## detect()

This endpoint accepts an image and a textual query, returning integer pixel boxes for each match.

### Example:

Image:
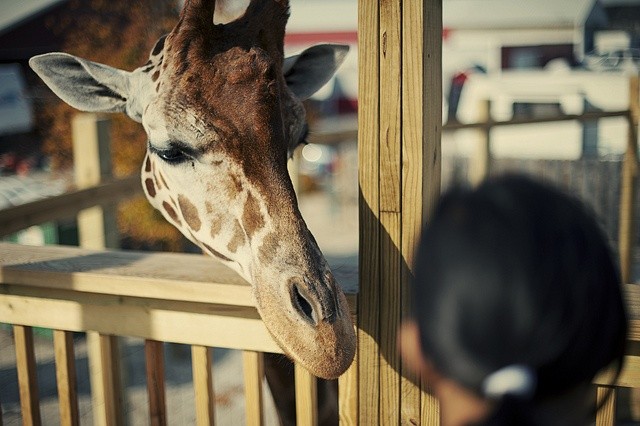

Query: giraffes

[0,0,355,426]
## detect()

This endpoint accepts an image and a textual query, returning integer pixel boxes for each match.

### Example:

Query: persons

[397,169,628,423]
